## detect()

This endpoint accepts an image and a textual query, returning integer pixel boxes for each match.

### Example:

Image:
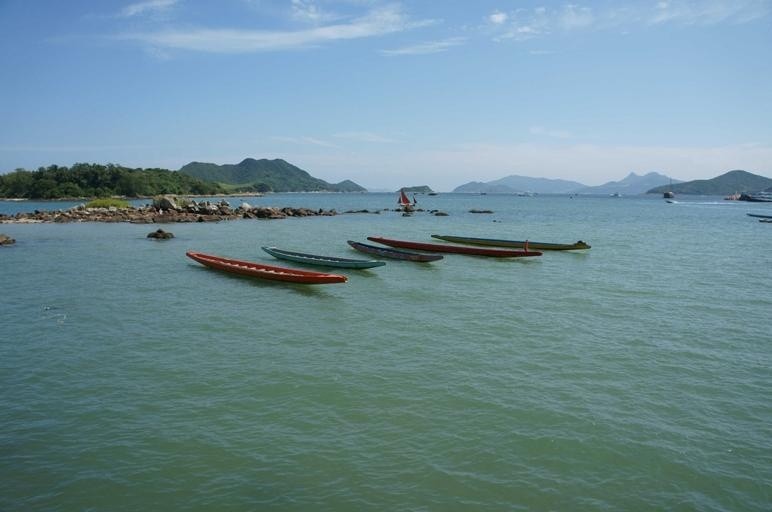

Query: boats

[366,235,543,261]
[259,244,387,271]
[431,233,591,253]
[184,248,349,286]
[345,240,444,264]
[663,191,674,198]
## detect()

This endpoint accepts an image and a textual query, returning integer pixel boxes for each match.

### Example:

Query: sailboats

[397,188,417,206]
